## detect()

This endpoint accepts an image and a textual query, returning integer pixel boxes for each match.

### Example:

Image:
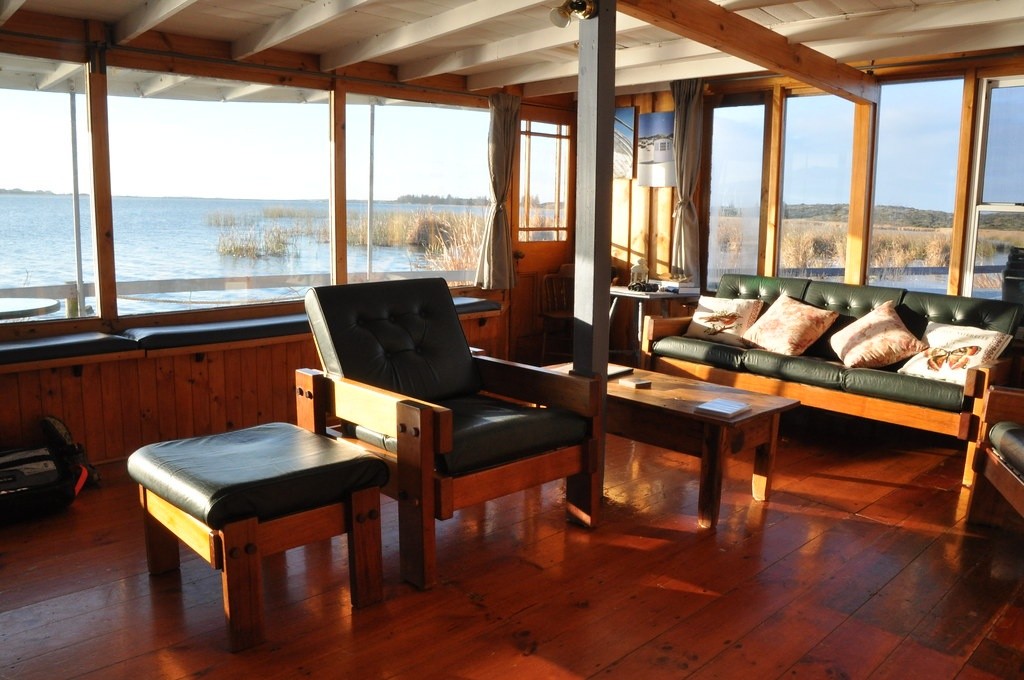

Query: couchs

[637,272,1024,488]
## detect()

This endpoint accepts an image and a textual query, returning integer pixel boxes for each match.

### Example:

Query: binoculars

[627,281,658,292]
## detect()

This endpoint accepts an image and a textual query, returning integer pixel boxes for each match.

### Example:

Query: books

[695,397,753,419]
[619,378,652,389]
[549,362,634,379]
[648,278,700,294]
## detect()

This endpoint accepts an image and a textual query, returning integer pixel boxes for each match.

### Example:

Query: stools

[126,420,390,658]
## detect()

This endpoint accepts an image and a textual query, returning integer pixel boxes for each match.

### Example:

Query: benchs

[1,292,502,482]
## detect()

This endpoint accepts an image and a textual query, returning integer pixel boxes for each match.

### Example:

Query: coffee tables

[539,359,803,529]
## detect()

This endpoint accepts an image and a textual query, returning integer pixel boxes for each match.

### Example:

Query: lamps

[548,0,599,28]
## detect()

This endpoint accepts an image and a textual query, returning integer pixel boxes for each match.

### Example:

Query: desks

[609,284,702,368]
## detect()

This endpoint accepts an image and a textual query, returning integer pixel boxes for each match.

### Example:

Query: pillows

[683,294,765,349]
[738,293,841,357]
[826,299,931,369]
[896,320,1014,386]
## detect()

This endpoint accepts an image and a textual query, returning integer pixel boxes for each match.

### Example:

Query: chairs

[952,385,1024,526]
[539,263,618,365]
[295,275,602,582]
